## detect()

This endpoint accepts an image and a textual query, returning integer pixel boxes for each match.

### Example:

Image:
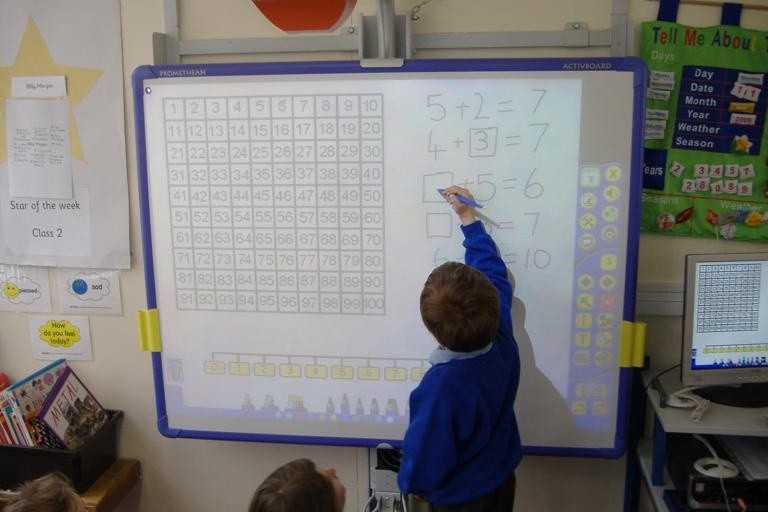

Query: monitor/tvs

[681,252,768,409]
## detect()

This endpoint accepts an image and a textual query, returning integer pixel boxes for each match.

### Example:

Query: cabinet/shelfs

[636,363,768,512]
[0,459,141,512]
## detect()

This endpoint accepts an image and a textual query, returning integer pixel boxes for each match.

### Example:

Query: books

[0,359,108,453]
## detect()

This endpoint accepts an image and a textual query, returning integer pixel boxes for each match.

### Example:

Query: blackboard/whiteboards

[134,58,648,460]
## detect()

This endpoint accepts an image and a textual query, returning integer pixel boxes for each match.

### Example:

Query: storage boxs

[0,408,124,493]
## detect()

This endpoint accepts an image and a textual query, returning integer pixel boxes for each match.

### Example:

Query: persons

[396,186,523,511]
[0,473,88,512]
[248,456,346,512]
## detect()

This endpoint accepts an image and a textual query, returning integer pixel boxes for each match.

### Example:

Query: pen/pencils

[436,188,484,209]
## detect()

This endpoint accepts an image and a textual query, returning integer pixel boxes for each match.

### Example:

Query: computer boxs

[664,435,749,512]
